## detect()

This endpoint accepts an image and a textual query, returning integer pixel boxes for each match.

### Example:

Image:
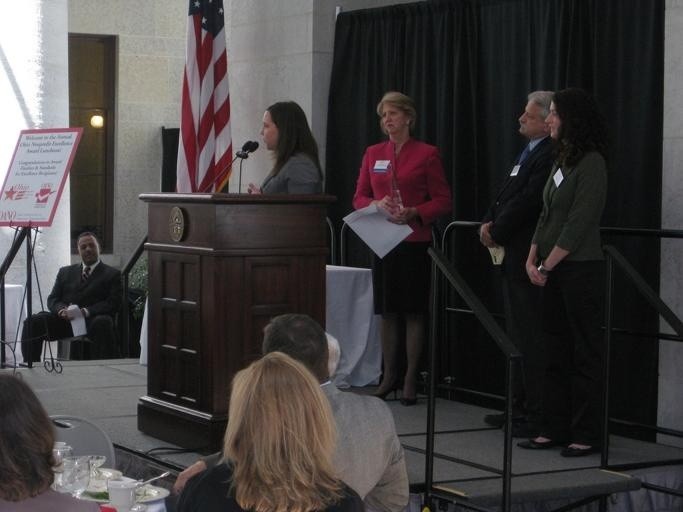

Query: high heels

[371,377,400,399]
[400,378,418,406]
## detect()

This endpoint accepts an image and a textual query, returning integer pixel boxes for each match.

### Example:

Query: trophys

[387,162,404,218]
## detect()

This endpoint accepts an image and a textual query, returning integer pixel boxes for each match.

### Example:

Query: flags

[176,0,232,193]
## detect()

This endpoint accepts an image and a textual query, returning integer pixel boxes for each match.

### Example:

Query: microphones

[200,141,253,192]
[239,141,260,195]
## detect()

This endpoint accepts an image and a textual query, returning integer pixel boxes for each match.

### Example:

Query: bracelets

[537,263,554,276]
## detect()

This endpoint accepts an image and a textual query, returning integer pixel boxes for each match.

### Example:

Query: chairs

[44,312,119,362]
[48,415,116,468]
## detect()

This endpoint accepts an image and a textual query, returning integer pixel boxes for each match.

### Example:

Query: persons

[258,316,409,512]
[21,231,122,361]
[516,89,611,458]
[353,91,451,408]
[247,102,323,196]
[1,374,102,511]
[173,332,341,495]
[480,89,558,437]
[172,352,365,511]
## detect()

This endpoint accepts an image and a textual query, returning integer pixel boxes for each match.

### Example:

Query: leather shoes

[561,442,598,458]
[485,408,524,426]
[517,434,559,450]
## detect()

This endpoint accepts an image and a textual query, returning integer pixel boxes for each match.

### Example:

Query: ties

[81,267,91,282]
[518,145,530,163]
